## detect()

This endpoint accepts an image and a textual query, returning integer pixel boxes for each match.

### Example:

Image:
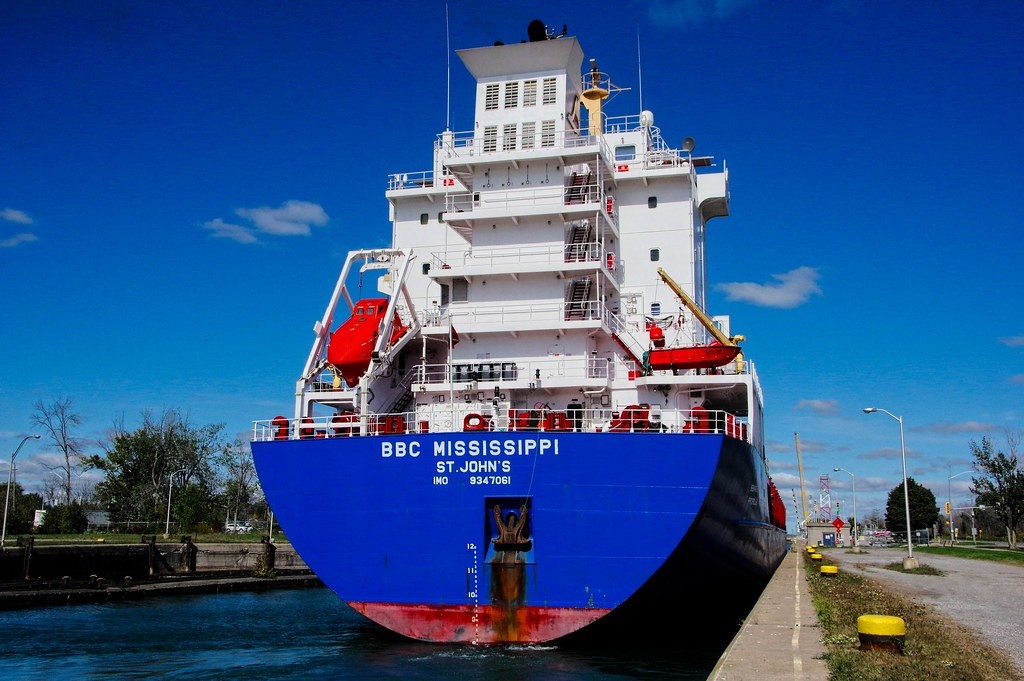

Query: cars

[247,527,253,533]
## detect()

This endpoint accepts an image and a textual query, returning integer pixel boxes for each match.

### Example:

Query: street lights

[164,469,188,538]
[948,470,975,541]
[834,468,858,547]
[863,407,912,557]
[2,434,40,544]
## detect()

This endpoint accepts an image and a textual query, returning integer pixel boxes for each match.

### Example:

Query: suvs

[227,524,247,533]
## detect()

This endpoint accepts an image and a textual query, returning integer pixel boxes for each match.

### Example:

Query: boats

[650,345,743,369]
[327,298,406,387]
[248,3,787,650]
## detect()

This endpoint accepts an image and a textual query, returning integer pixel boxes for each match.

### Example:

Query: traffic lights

[946,502,951,514]
